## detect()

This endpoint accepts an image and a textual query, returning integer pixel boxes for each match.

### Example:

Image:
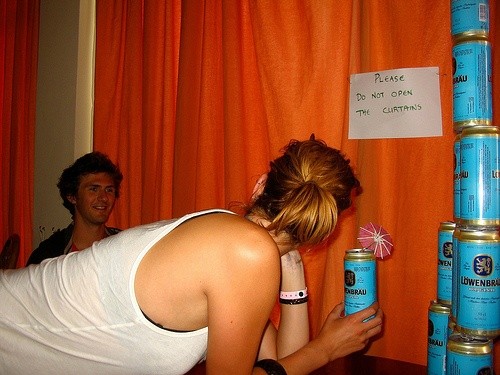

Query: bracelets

[279,287,308,300]
[279,296,308,304]
[254,359,287,375]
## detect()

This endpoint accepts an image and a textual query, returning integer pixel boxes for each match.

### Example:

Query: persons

[0,139,383,375]
[27,152,122,269]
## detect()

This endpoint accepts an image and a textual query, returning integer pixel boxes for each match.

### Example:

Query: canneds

[343,248,377,323]
[449,0,492,131]
[427,126,500,375]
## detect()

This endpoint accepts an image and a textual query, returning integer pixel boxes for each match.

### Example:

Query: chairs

[0,233,20,270]
